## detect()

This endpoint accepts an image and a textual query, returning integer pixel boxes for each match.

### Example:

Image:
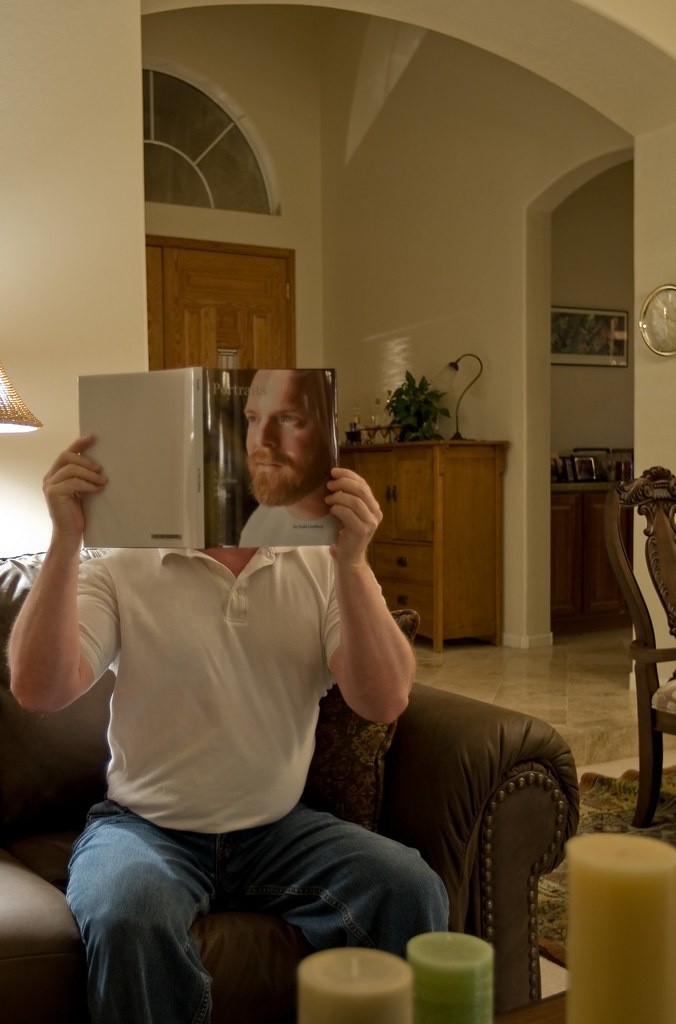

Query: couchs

[0,549,583,1024]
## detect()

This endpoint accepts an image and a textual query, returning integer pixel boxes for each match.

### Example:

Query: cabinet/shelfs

[550,481,634,637]
[338,437,507,653]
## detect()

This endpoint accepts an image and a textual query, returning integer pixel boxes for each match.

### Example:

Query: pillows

[304,608,423,830]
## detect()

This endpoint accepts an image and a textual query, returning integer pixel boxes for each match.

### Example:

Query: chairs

[601,462,676,830]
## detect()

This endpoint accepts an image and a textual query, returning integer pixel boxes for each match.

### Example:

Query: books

[74,367,340,549]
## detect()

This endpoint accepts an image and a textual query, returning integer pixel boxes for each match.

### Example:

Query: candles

[405,930,501,1024]
[296,946,415,1024]
[561,833,676,1024]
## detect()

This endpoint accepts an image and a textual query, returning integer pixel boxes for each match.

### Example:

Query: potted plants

[382,369,451,442]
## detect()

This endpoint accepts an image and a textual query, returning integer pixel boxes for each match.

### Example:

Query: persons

[240,369,339,546]
[9,433,450,1024]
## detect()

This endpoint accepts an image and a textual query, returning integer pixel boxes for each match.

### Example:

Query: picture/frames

[551,305,629,368]
[553,445,635,481]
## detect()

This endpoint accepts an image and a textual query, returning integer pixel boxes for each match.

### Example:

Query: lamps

[0,361,47,434]
[434,352,485,441]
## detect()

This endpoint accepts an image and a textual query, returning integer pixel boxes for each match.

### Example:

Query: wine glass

[364,414,402,445]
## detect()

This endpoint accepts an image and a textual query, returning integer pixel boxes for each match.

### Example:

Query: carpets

[536,762,676,973]
